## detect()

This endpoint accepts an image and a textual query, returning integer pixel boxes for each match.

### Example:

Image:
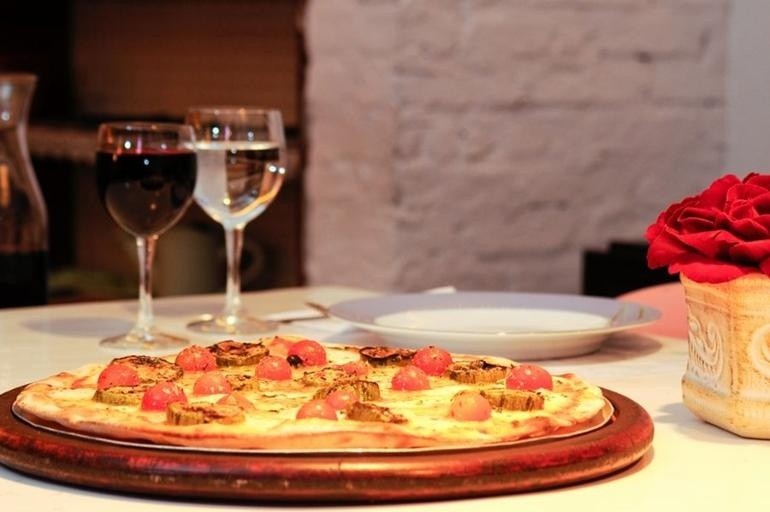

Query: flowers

[645,168,770,283]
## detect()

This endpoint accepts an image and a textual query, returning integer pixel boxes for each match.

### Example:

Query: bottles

[0,74,51,305]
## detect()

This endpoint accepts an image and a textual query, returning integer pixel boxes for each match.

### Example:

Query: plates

[327,288,664,361]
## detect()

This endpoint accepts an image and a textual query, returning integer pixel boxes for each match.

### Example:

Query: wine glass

[182,107,287,338]
[93,122,199,352]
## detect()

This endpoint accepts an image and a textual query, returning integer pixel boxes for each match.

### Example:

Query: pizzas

[13,332,607,452]
